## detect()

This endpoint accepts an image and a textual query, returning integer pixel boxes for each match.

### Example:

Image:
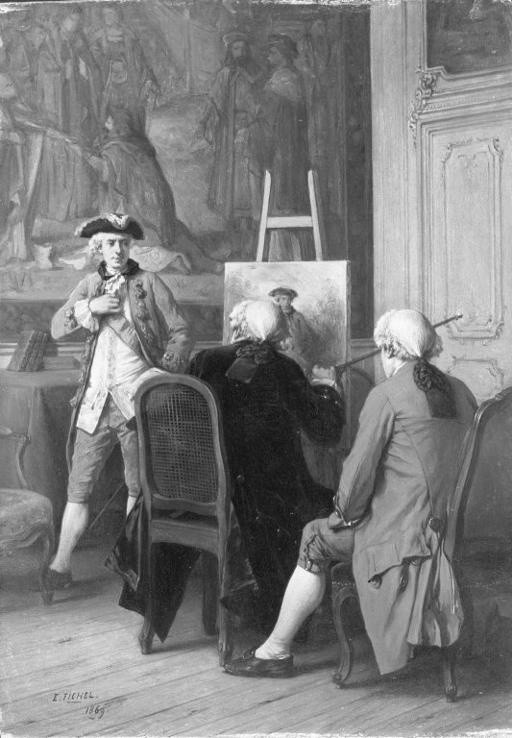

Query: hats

[75,212,143,239]
[268,287,298,297]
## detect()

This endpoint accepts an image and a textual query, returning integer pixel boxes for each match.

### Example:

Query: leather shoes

[32,568,72,592]
[224,648,293,677]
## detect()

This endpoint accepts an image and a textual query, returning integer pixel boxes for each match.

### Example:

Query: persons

[32,1,105,158]
[104,292,347,640]
[0,36,78,265]
[86,0,162,136]
[189,29,271,229]
[223,301,480,677]
[71,106,226,278]
[269,286,317,381]
[21,210,195,593]
[240,31,327,263]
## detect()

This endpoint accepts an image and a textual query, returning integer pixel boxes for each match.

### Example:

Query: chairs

[133,372,266,667]
[0,426,56,607]
[327,387,511,702]
